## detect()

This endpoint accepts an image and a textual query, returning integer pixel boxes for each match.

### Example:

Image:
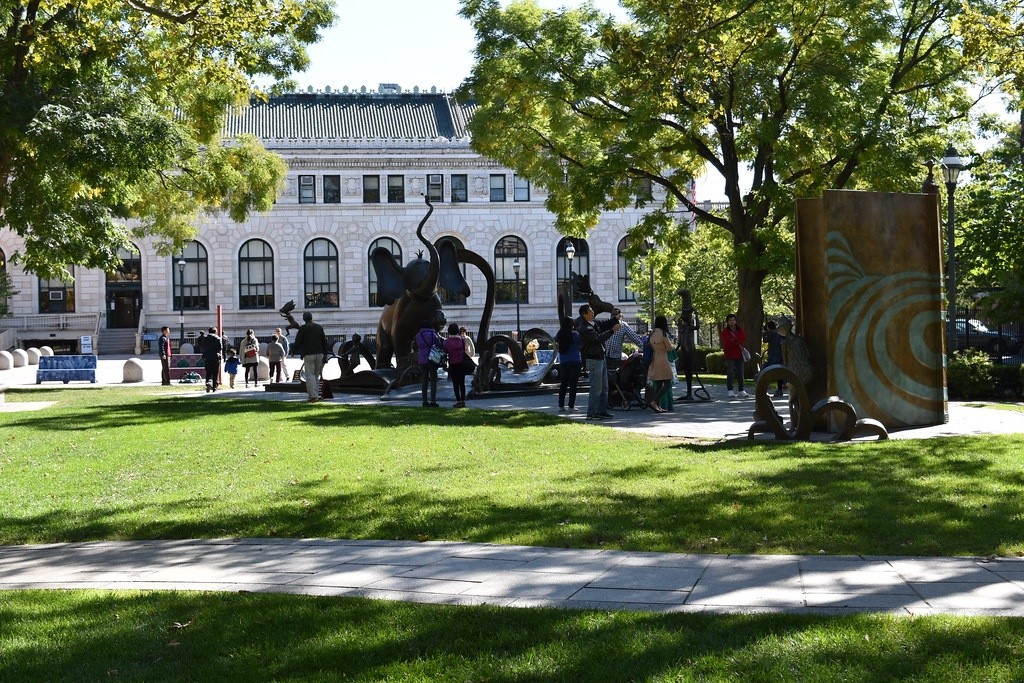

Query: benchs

[167,353,222,380]
[36,355,98,383]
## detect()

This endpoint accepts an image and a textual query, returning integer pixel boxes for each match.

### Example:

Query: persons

[773,312,814,432]
[195,330,206,353]
[578,304,624,420]
[239,328,261,388]
[720,314,749,398]
[276,328,290,382]
[280,312,301,336]
[291,312,328,402]
[416,319,475,407]
[225,349,241,389]
[265,335,285,384]
[201,326,223,394]
[555,315,582,411]
[645,315,679,413]
[222,331,229,363]
[524,337,539,366]
[763,321,786,399]
[342,333,361,377]
[603,309,644,374]
[158,326,172,385]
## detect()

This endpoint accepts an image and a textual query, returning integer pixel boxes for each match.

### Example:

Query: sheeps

[555,289,613,337]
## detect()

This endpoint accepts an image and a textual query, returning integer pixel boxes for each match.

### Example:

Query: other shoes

[213,388,216,393]
[558,407,566,412]
[207,385,210,393]
[567,407,579,412]
[771,392,783,398]
[453,401,465,407]
[430,402,439,407]
[660,408,667,412]
[423,402,430,407]
[308,398,319,403]
[599,412,612,419]
[586,413,603,420]
[650,402,662,412]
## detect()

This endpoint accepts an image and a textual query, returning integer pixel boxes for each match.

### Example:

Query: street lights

[565,240,576,319]
[939,141,960,367]
[644,224,657,330]
[511,257,521,341]
[177,256,187,348]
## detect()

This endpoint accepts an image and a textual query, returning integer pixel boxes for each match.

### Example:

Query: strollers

[606,350,648,411]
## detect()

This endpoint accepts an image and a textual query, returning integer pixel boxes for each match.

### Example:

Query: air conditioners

[49,291,63,300]
[301,176,313,185]
[430,174,441,184]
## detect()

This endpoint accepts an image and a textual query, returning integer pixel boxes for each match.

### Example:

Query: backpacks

[245,338,257,357]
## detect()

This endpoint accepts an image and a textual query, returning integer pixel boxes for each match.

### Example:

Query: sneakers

[738,391,749,397]
[728,391,735,397]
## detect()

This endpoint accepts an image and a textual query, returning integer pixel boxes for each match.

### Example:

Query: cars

[944,318,1024,357]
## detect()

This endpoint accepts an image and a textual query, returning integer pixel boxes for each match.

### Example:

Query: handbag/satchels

[427,344,441,364]
[741,347,751,362]
[643,335,653,363]
[461,353,475,374]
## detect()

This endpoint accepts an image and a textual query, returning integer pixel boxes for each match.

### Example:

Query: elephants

[371,195,470,379]
